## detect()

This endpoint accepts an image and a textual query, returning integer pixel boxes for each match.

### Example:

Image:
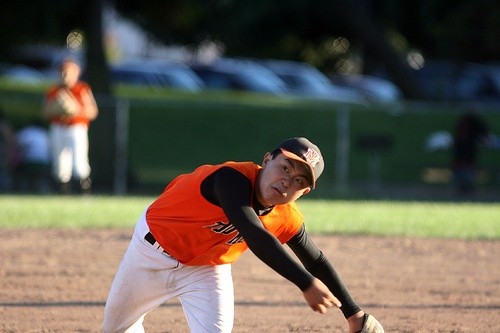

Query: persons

[0,26,493,195]
[101,137,384,333]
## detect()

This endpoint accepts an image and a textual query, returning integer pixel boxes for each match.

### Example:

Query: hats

[279,136,325,189]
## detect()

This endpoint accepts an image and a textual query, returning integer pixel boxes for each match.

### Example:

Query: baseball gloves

[56,86,80,117]
